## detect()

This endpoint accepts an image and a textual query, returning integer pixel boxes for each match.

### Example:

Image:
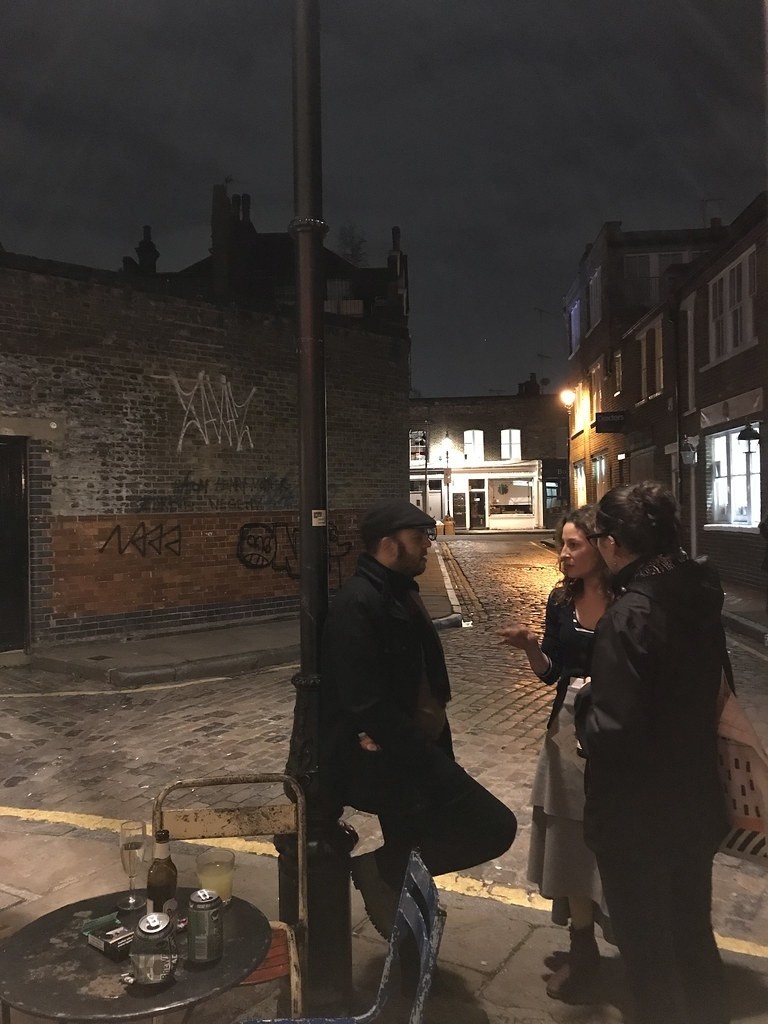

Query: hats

[358,497,436,549]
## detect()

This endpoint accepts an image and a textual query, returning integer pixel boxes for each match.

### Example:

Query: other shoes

[545,934,602,998]
[351,852,405,943]
[402,971,444,997]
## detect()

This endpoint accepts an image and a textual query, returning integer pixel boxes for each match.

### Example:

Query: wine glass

[115,820,146,911]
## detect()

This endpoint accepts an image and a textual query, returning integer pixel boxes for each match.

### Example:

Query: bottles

[147,831,178,938]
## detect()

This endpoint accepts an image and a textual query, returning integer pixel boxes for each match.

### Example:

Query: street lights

[441,432,453,520]
[560,389,576,517]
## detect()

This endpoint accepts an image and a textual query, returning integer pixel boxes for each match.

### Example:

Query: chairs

[235,847,447,1024]
[151,772,307,1023]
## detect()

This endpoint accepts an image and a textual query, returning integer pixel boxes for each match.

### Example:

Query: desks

[0,887,273,1024]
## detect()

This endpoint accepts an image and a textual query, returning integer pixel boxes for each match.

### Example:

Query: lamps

[680,433,696,465]
[737,419,768,454]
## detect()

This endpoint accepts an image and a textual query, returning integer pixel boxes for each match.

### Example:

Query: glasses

[586,533,620,550]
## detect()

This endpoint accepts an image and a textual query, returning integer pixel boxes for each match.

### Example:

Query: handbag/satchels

[713,689,768,868]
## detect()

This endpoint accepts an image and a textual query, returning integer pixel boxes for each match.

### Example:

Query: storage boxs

[87,924,135,963]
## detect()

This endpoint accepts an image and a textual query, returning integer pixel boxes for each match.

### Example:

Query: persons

[573,481,737,1024]
[495,505,618,1001]
[284,499,518,944]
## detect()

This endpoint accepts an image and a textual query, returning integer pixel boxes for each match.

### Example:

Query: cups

[194,850,234,905]
